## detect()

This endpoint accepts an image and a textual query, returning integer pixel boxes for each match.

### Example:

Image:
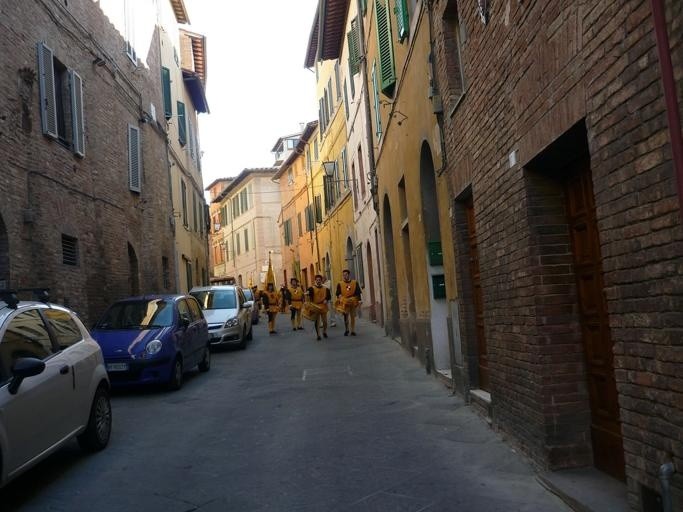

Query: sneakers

[323,333,328,338]
[318,336,321,340]
[351,332,356,336]
[344,331,349,336]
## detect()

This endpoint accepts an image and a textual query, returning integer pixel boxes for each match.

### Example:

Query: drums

[334,296,351,315]
[302,303,321,322]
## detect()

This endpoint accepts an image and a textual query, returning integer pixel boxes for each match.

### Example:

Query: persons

[337,270,362,336]
[251,275,337,341]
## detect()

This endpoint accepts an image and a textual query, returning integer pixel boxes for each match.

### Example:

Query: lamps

[320,157,359,195]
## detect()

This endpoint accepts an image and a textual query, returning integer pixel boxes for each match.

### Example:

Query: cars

[91,293,211,390]
[189,285,259,350]
[0,299,112,485]
